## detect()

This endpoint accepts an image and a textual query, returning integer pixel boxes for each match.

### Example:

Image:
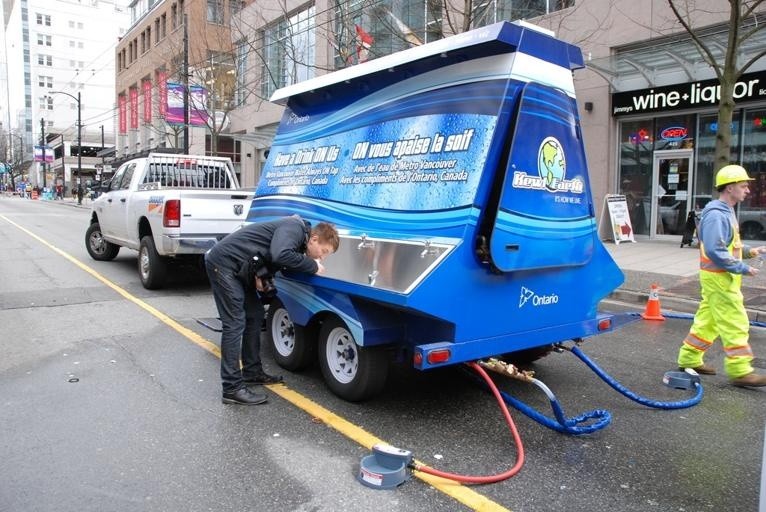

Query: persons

[677,164,766,388]
[0,161,106,207]
[203,214,341,406]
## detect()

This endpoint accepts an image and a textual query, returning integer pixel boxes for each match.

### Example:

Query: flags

[353,23,374,65]
[396,19,425,49]
[324,35,359,65]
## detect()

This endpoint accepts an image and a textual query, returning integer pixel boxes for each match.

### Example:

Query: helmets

[715,163,756,188]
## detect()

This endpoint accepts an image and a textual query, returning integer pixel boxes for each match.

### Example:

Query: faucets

[420,239,441,262]
[356,233,375,257]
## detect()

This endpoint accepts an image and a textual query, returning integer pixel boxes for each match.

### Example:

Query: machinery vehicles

[196,19,706,489]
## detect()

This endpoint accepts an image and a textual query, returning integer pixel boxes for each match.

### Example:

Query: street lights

[47,89,82,208]
[7,133,25,181]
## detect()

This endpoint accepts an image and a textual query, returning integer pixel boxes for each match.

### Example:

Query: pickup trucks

[86,154,254,290]
[660,196,766,239]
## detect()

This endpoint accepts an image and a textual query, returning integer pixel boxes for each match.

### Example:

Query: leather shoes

[240,370,286,385]
[221,387,268,406]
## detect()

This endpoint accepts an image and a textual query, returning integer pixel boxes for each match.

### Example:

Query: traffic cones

[639,282,665,322]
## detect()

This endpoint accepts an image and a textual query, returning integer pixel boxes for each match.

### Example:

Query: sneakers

[732,373,766,387]
[678,362,717,375]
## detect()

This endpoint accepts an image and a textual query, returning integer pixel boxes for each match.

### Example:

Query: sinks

[308,234,456,296]
